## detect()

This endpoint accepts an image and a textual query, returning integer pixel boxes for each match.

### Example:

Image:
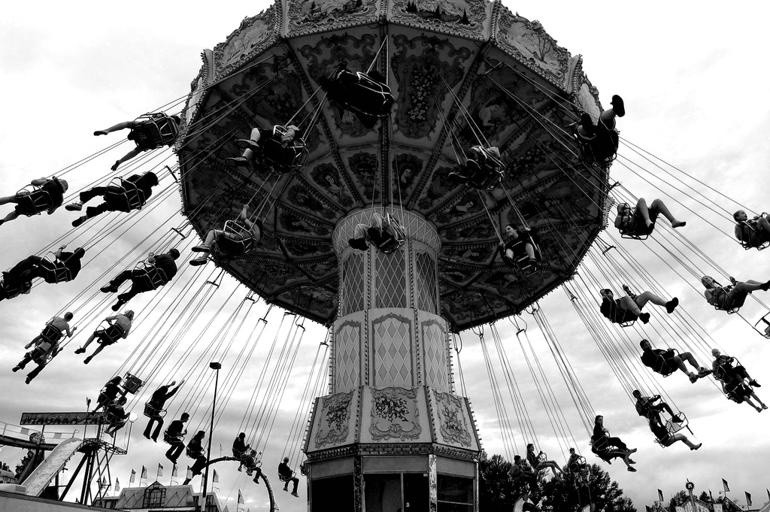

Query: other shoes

[237,467,298,498]
[90,409,202,476]
[12,346,92,385]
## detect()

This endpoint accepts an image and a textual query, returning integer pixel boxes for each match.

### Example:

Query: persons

[92,113,182,170]
[598,285,679,324]
[222,123,300,170]
[735,209,770,249]
[572,94,625,166]
[190,204,262,265]
[498,224,541,275]
[0,177,68,226]
[615,199,686,237]
[701,276,770,310]
[448,143,507,190]
[65,172,159,227]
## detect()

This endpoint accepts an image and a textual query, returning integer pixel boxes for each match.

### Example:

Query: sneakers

[762,280,770,291]
[112,160,119,171]
[647,220,654,230]
[581,110,596,135]
[112,299,123,312]
[189,257,208,265]
[236,136,262,152]
[93,129,108,136]
[528,415,703,493]
[65,203,82,211]
[101,285,117,294]
[641,312,651,324]
[612,94,625,118]
[448,171,469,184]
[748,379,760,387]
[528,258,538,268]
[224,156,250,169]
[699,369,714,378]
[671,220,686,229]
[666,296,680,313]
[466,158,483,176]
[192,244,212,254]
[502,256,518,271]
[72,216,87,227]
[689,372,700,382]
[756,402,768,412]
